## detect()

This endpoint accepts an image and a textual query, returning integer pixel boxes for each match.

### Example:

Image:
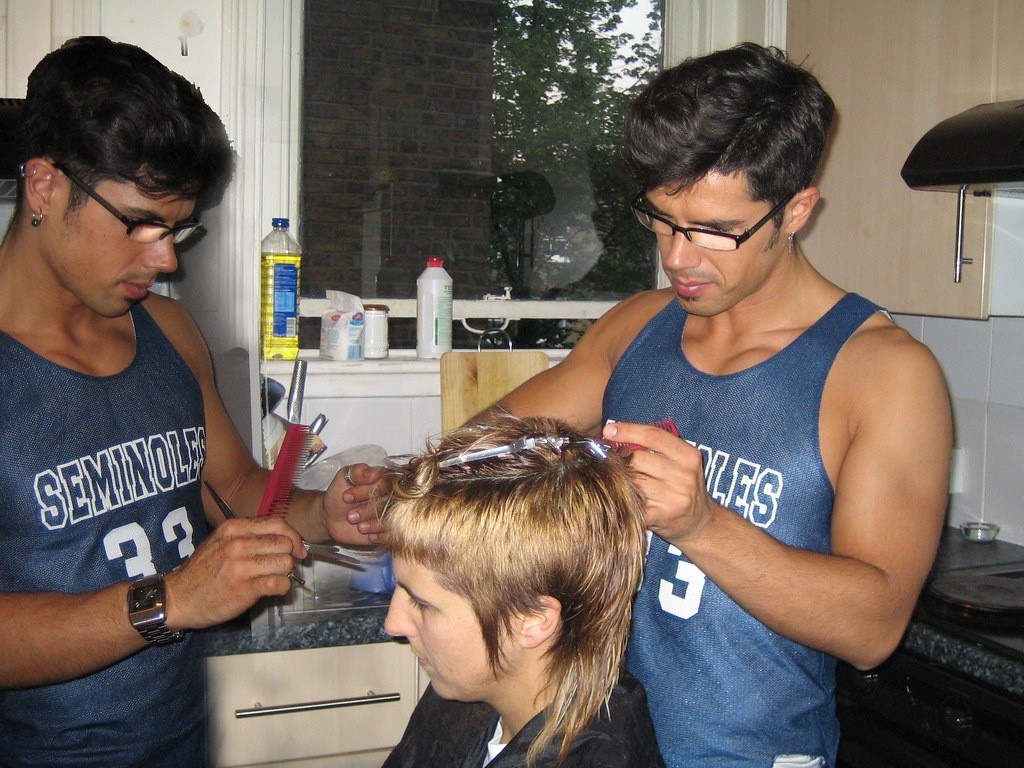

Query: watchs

[127,574,184,646]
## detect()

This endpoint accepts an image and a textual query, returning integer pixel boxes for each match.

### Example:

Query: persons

[458,43,952,767]
[380,412,664,768]
[0,36,395,768]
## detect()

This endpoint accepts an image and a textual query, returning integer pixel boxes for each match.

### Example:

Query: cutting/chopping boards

[439,329,549,438]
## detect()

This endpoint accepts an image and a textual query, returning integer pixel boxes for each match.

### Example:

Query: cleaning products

[416,258,453,360]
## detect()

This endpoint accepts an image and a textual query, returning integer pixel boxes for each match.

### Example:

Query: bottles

[415,256,455,361]
[260,217,302,361]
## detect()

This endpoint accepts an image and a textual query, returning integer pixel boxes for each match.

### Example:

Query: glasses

[53,159,203,246]
[631,184,811,253]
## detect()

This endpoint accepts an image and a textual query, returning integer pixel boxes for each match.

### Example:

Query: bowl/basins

[960,522,1000,544]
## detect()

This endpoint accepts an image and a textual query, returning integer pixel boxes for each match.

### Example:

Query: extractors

[899,99,1024,284]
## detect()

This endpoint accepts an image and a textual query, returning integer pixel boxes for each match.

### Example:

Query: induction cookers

[836,569,1024,768]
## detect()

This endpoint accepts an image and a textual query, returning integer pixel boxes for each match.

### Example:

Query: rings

[344,465,358,486]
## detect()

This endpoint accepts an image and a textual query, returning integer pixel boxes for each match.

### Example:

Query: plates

[931,569,1024,616]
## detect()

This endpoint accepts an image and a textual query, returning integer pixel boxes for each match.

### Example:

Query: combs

[259,425,315,519]
[602,419,679,456]
